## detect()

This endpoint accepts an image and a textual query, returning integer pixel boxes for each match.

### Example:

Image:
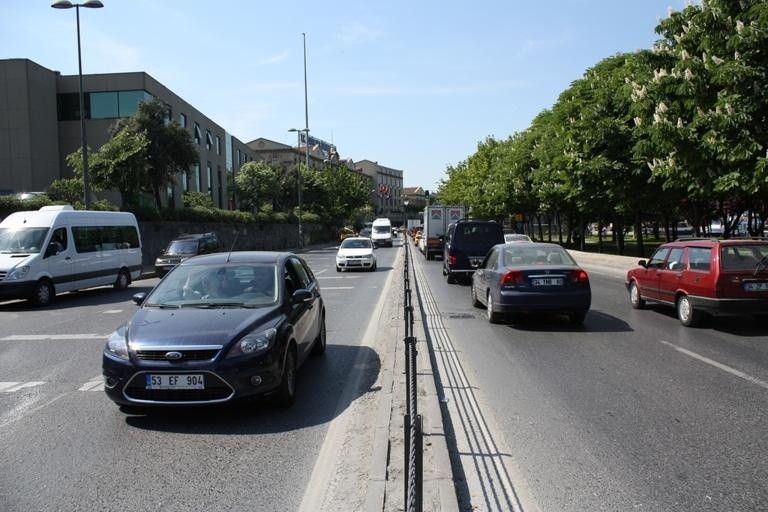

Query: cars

[101,250,327,411]
[589,216,762,236]
[335,237,378,273]
[471,242,591,325]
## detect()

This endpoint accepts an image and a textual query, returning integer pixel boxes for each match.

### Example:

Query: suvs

[154,233,220,280]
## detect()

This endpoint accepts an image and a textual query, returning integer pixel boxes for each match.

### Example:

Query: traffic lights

[425,190,429,199]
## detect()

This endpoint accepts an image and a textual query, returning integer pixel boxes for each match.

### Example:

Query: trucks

[356,218,398,249]
[408,206,536,284]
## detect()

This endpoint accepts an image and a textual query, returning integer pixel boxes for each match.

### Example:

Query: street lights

[50,1,104,211]
[288,128,310,250]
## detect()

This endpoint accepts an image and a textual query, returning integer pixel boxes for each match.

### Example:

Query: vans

[625,237,768,327]
[0,204,143,307]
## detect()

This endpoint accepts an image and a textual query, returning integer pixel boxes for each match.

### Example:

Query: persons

[194,266,233,301]
[239,266,282,301]
[40,232,65,252]
